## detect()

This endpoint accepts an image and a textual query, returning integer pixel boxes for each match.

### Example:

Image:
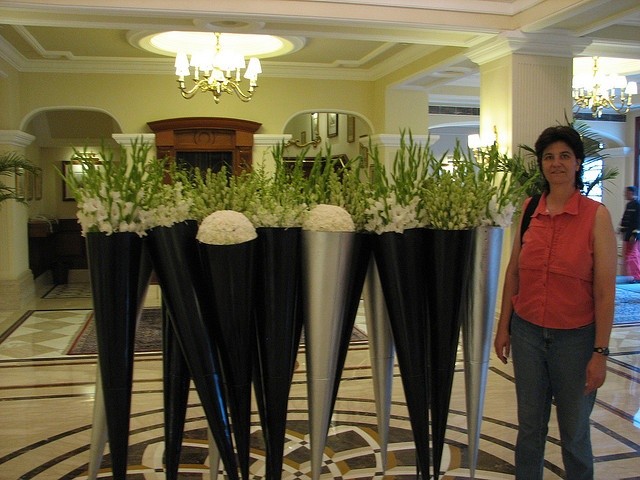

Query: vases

[87,238,156,480]
[427,229,475,480]
[83,232,142,479]
[461,227,503,479]
[161,289,190,480]
[194,236,256,480]
[362,252,394,475]
[146,220,240,480]
[249,227,304,480]
[327,230,376,432]
[304,232,372,479]
[372,228,430,479]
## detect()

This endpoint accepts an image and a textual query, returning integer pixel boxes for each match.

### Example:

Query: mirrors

[282,109,375,195]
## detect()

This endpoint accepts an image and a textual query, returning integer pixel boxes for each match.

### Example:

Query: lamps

[571,56,639,119]
[466,125,497,164]
[174,32,264,104]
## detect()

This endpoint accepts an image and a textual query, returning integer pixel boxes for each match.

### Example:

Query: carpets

[610,274,640,327]
[60,308,370,355]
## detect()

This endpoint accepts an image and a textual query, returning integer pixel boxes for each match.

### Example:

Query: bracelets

[593,347,610,356]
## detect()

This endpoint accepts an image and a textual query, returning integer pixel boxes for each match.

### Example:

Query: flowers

[299,139,373,232]
[49,136,150,239]
[298,204,355,232]
[257,137,308,227]
[448,139,541,229]
[193,209,258,245]
[362,126,450,234]
[190,158,274,227]
[420,169,499,232]
[140,153,196,233]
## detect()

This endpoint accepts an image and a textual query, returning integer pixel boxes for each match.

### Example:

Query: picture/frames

[27,166,34,201]
[35,170,44,200]
[327,113,338,138]
[346,115,355,143]
[311,112,319,141]
[15,165,27,200]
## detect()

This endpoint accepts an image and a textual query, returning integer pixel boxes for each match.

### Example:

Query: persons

[616,186,640,282]
[493,126,617,480]
